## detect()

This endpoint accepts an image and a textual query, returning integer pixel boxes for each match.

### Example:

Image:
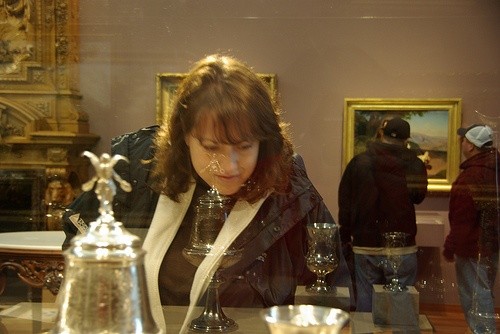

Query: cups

[467,252,500,334]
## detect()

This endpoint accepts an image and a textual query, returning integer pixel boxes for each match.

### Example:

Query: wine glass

[305,222,340,294]
[383,231,409,292]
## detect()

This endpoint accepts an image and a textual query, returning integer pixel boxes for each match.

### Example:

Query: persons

[63,55,352,306]
[338,119,429,312]
[442,125,500,334]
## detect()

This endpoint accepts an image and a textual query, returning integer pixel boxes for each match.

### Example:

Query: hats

[456,124,494,148]
[384,119,411,139]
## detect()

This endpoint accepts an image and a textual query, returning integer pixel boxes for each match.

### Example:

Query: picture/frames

[342,98,463,193]
[154,72,278,126]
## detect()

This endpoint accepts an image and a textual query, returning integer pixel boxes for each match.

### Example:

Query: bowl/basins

[258,303,351,334]
